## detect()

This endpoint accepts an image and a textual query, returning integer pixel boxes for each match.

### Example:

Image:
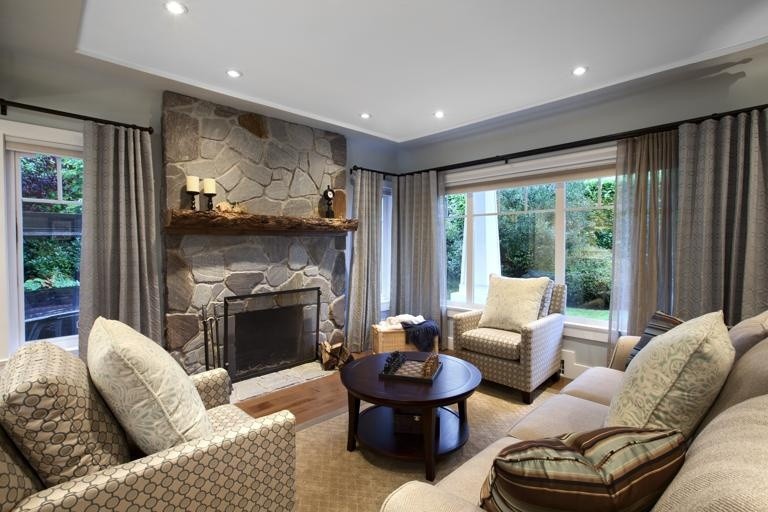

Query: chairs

[0,313,298,512]
[453,273,570,404]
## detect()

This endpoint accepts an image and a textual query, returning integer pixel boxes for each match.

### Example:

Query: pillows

[607,309,737,440]
[479,273,554,334]
[623,310,686,370]
[480,428,689,512]
[83,314,220,459]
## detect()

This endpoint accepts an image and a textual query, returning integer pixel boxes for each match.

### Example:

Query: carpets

[277,385,565,512]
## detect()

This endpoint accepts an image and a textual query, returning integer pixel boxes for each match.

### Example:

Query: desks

[339,351,473,482]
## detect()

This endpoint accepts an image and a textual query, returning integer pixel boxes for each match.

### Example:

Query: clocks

[323,184,335,218]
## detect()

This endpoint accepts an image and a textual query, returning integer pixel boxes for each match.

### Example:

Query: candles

[185,175,217,194]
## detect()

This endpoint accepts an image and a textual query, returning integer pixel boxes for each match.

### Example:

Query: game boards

[378,359,442,384]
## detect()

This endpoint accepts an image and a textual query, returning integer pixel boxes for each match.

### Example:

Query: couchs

[377,307,768,512]
